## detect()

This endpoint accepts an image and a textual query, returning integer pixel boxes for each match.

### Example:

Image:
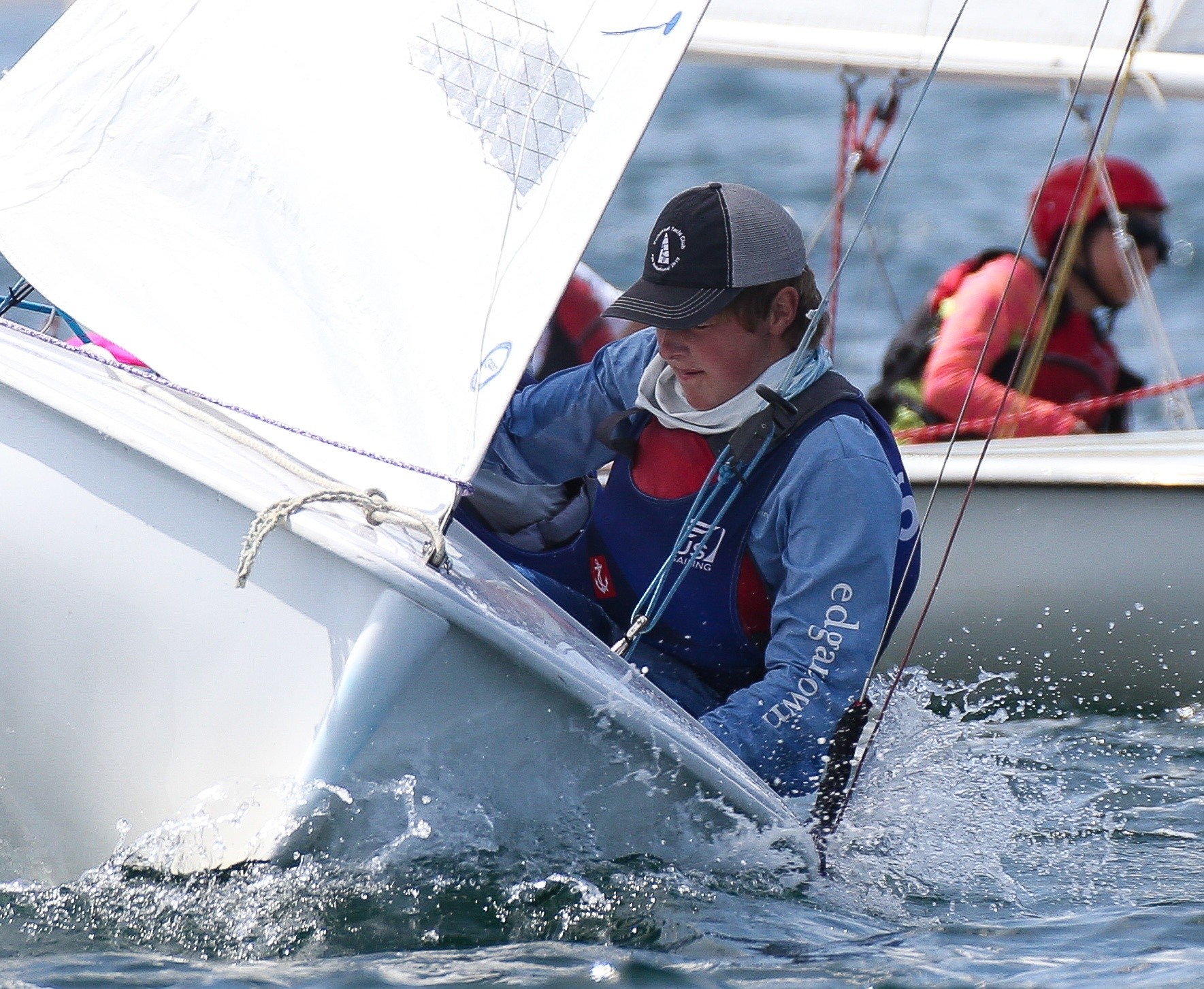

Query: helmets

[1030,154,1167,258]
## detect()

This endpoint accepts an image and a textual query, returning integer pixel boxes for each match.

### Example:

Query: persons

[455,181,920,796]
[866,153,1172,446]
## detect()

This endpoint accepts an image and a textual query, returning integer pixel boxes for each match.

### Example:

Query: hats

[600,183,807,331]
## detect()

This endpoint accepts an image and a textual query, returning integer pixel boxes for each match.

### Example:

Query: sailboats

[670,0,1203,719]
[1,0,834,902]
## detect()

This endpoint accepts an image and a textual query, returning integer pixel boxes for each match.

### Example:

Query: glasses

[1102,217,1171,266]
